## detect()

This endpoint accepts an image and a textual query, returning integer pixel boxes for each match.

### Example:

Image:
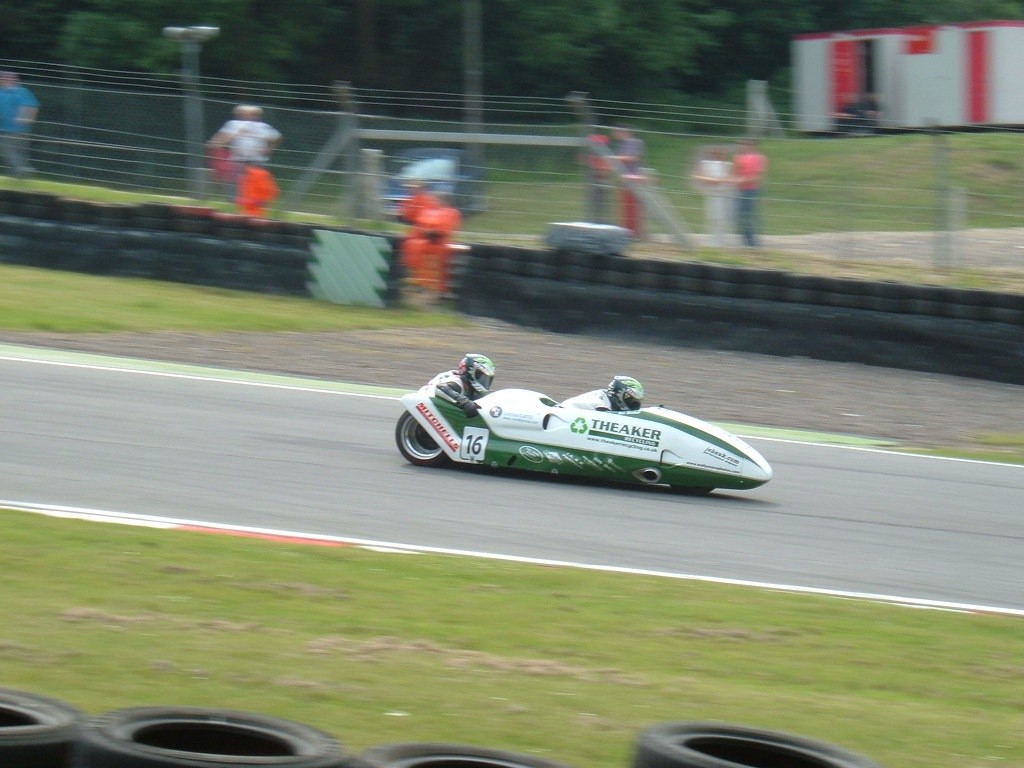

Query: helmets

[459,353,495,396]
[606,375,644,411]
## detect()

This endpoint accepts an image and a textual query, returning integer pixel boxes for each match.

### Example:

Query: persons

[550,375,646,413]
[690,134,770,246]
[586,125,646,238]
[830,92,883,137]
[209,103,284,208]
[396,183,438,286]
[423,352,496,417]
[0,68,39,178]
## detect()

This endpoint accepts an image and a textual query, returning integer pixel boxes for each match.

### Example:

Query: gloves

[456,397,482,418]
